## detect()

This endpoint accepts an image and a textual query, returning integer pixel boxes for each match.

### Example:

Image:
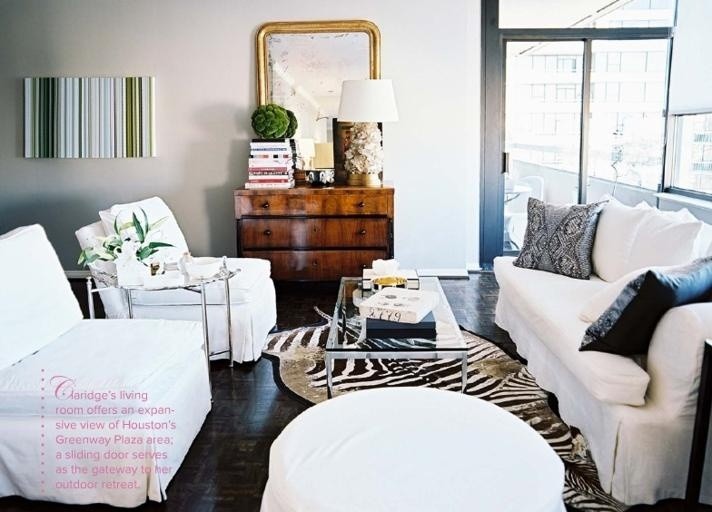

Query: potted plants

[76,205,176,286]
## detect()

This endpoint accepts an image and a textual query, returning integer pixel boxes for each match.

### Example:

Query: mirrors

[256,19,383,181]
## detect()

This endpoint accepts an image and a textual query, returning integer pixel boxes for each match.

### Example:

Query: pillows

[579,257,712,353]
[513,197,608,280]
[99,197,188,271]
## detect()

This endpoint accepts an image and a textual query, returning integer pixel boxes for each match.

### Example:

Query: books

[358,286,439,340]
[242,137,297,190]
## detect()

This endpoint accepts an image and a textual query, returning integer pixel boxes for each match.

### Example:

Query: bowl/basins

[372,277,408,295]
[184,256,222,278]
[304,169,336,186]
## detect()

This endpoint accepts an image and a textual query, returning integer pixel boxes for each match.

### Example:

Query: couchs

[492,196,712,508]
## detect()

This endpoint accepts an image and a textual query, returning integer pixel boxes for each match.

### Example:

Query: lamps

[337,78,397,189]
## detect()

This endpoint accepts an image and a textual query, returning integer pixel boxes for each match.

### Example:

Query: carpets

[259,314,624,510]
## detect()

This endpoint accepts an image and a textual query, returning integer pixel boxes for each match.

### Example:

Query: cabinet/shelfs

[235,181,397,285]
